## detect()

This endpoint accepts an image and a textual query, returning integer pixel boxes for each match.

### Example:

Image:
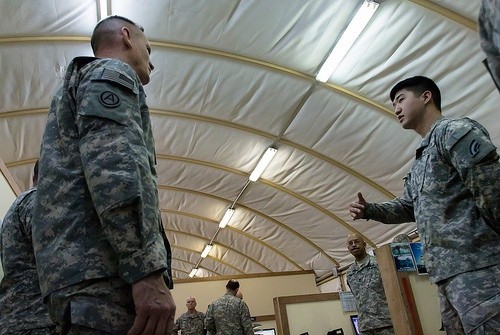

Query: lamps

[248,147,278,182]
[201,244,212,258]
[189,268,198,278]
[315,0,380,83]
[219,209,235,229]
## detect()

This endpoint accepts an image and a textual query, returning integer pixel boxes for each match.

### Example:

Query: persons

[206,279,254,335]
[33,16,176,335]
[0,163,55,335]
[477,0,500,90]
[346,234,394,335]
[348,75,500,335]
[173,297,207,335]
